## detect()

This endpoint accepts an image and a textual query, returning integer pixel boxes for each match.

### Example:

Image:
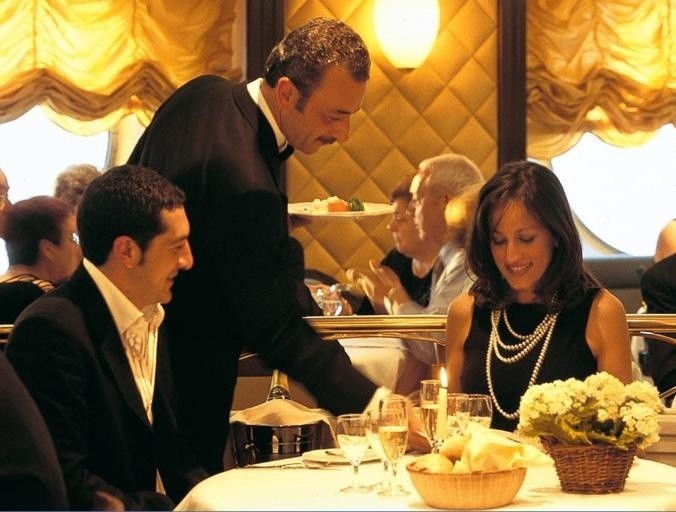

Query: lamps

[374,0,439,71]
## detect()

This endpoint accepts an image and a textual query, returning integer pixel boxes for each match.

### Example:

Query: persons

[6,166,196,511]
[345,177,437,315]
[357,153,486,400]
[0,162,102,339]
[398,161,632,454]
[127,13,391,506]
[640,217,676,407]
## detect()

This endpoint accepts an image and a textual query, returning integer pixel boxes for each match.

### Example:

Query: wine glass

[335,396,412,497]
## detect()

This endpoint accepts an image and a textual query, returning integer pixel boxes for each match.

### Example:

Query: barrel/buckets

[232,421,322,468]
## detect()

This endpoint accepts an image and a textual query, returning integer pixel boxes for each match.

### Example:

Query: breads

[414,435,524,474]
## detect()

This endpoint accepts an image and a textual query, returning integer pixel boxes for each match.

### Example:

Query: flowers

[516,372,661,446]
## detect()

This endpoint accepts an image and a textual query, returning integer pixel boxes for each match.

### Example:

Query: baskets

[540,436,644,495]
[406,453,527,510]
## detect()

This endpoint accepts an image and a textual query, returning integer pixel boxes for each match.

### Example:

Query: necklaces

[485,287,563,420]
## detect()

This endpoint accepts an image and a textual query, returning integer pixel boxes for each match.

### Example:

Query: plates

[286,201,397,219]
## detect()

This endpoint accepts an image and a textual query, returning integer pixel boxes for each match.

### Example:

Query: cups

[313,291,344,318]
[298,363,494,471]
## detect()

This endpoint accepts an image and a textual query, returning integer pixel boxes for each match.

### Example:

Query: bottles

[264,368,290,402]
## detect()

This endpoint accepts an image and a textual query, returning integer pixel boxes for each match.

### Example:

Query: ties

[432,258,445,293]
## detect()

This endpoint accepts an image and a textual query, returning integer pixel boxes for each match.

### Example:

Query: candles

[435,366,452,442]
